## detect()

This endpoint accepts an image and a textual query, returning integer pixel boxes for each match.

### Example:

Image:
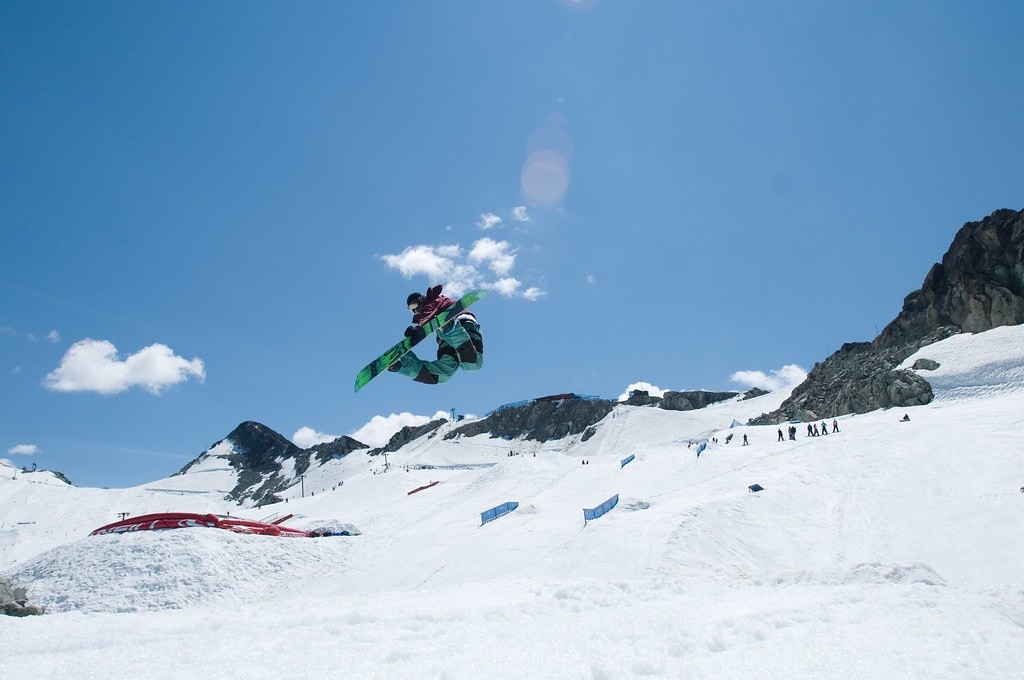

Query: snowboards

[352,288,490,393]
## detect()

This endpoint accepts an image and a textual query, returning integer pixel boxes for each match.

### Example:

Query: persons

[833,419,840,432]
[788,426,796,440]
[903,414,909,420]
[808,423,820,436]
[387,292,483,384]
[778,429,784,441]
[821,421,828,435]
[743,434,749,446]
[510,450,512,456]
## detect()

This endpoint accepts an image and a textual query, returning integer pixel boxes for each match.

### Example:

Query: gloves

[404,326,415,337]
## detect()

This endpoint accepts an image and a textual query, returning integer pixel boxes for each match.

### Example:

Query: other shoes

[443,316,458,335]
[387,360,402,372]
